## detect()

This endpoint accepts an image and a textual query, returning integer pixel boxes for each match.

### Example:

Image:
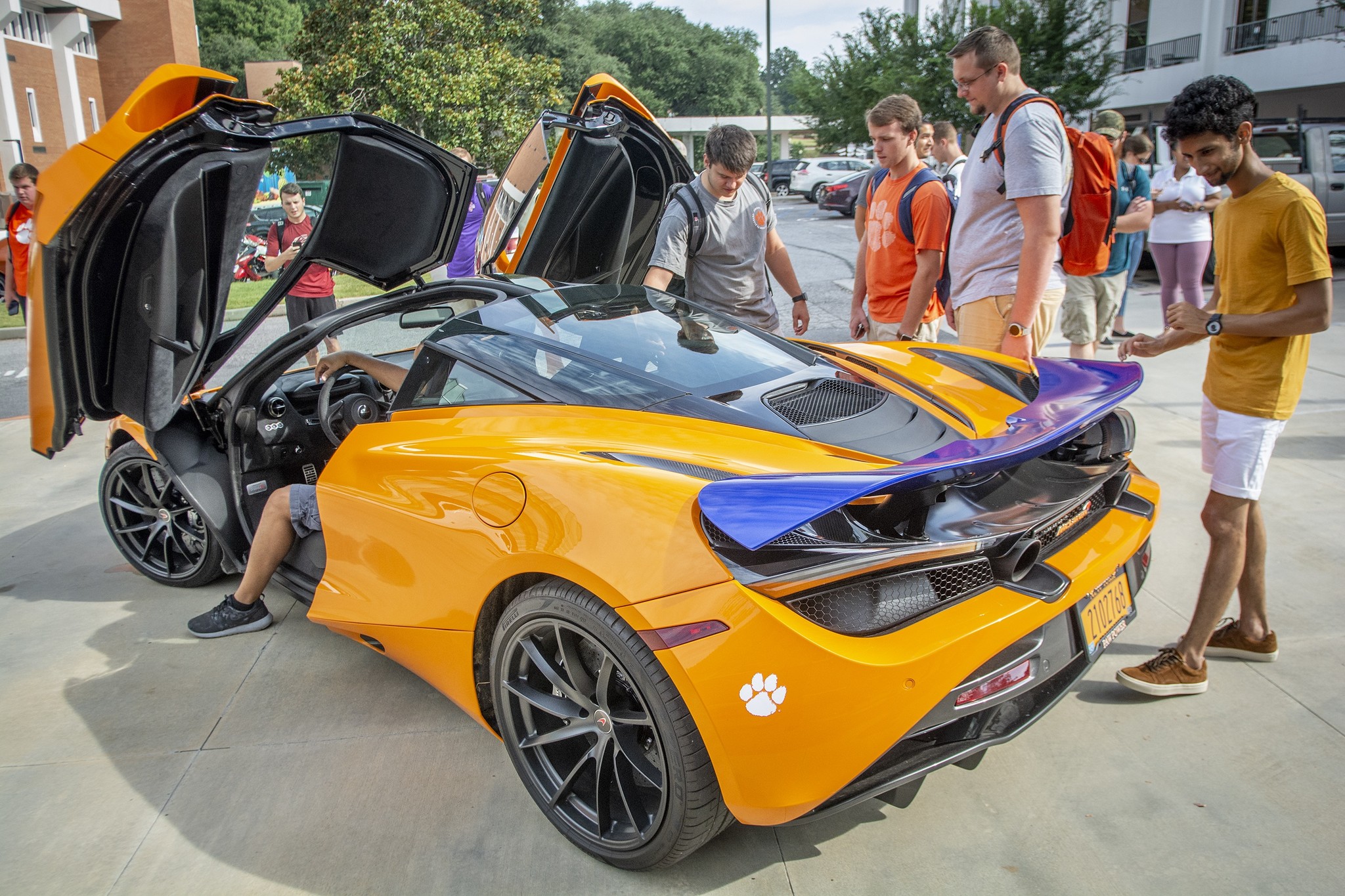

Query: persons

[642,127,813,351]
[644,288,742,355]
[853,117,973,251]
[263,183,342,369]
[1143,127,1223,331]
[1115,77,1331,698]
[849,93,954,350]
[1056,109,1158,377]
[0,163,46,333]
[445,147,496,279]
[188,329,468,640]
[936,26,1075,367]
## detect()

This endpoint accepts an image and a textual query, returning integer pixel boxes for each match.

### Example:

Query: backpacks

[870,167,951,310]
[979,93,1120,276]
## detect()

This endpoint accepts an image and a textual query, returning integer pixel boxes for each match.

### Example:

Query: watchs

[1004,321,1034,338]
[792,292,808,303]
[896,329,913,342]
[1205,313,1222,336]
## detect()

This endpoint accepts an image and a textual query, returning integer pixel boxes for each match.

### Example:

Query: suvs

[788,157,875,204]
[760,159,801,197]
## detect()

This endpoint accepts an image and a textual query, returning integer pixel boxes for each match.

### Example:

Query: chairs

[550,341,649,391]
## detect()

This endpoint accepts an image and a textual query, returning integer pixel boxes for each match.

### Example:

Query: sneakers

[1115,648,1208,696]
[186,592,274,638]
[1177,616,1279,662]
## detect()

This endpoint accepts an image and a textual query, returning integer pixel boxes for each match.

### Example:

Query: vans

[1203,122,1345,285]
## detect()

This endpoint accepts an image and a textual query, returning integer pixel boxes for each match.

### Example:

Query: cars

[240,201,323,242]
[749,161,767,175]
[816,168,872,218]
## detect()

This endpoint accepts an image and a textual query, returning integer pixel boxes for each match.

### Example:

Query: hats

[1093,109,1125,137]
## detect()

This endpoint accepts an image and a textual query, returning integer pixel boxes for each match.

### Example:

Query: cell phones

[8,301,19,315]
[293,242,301,251]
[1179,201,1192,208]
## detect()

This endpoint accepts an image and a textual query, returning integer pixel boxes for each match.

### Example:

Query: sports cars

[11,55,1167,872]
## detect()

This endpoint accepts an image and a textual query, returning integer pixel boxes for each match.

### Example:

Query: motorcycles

[232,222,285,283]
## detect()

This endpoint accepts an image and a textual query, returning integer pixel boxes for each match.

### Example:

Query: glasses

[1132,153,1147,164]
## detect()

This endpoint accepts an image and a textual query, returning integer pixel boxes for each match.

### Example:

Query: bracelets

[1199,204,1204,212]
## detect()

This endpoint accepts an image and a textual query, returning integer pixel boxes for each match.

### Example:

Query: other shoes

[1110,330,1135,339]
[1097,336,1114,348]
